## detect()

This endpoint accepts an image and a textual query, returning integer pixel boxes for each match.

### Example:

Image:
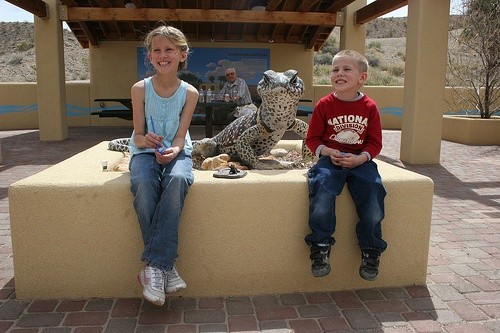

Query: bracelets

[234,96,237,101]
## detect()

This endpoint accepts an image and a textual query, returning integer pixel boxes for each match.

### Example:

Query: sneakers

[138,264,187,306]
[310,245,331,276]
[359,252,379,281]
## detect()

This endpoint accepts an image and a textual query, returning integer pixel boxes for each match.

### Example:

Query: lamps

[124,0,136,9]
[249,0,268,11]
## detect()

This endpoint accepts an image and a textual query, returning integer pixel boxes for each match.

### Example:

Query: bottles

[224,88,230,102]
[198,89,204,103]
[206,89,212,103]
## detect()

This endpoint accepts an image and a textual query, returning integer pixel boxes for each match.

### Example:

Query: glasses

[226,72,235,78]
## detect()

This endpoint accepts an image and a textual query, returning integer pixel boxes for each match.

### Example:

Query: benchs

[190,114,227,124]
[91,110,132,121]
[296,110,313,117]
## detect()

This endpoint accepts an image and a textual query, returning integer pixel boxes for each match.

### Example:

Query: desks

[253,95,313,102]
[94,98,132,110]
[193,102,237,138]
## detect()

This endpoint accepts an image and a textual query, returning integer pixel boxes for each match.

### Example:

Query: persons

[303,49,388,281]
[127,26,200,306]
[220,67,252,121]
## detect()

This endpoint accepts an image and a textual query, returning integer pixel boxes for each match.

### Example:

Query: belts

[236,103,251,107]
